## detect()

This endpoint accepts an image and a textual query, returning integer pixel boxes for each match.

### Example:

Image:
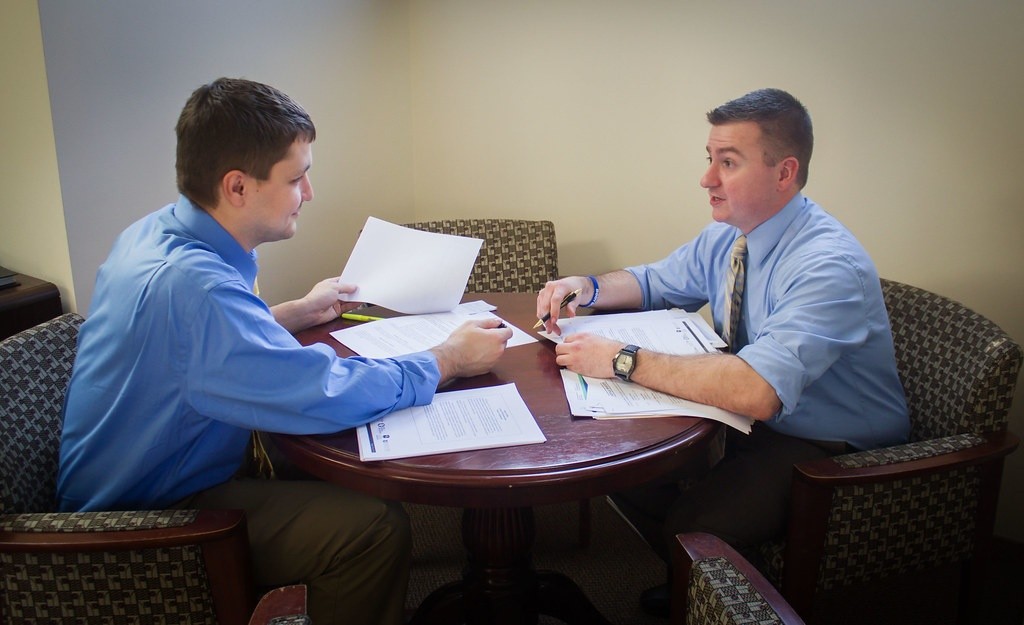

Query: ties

[251,276,274,480]
[705,235,747,469]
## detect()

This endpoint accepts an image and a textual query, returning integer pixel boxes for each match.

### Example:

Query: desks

[259,292,726,625]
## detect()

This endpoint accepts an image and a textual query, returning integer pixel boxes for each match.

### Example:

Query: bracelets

[580,275,600,309]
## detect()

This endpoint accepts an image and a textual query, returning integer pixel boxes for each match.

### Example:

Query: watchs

[611,344,640,383]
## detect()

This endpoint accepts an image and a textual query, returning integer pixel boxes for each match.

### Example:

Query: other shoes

[638,584,669,616]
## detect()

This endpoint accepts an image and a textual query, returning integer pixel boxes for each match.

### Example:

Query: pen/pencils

[341,313,385,322]
[531,288,584,329]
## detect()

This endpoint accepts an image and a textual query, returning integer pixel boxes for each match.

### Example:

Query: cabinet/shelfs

[0,265,64,343]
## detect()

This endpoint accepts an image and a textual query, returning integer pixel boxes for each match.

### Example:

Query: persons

[56,74,518,624]
[535,86,908,621]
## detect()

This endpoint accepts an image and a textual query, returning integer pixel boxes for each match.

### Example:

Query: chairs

[250,531,807,625]
[763,276,1024,625]
[398,219,559,295]
[0,312,252,625]
